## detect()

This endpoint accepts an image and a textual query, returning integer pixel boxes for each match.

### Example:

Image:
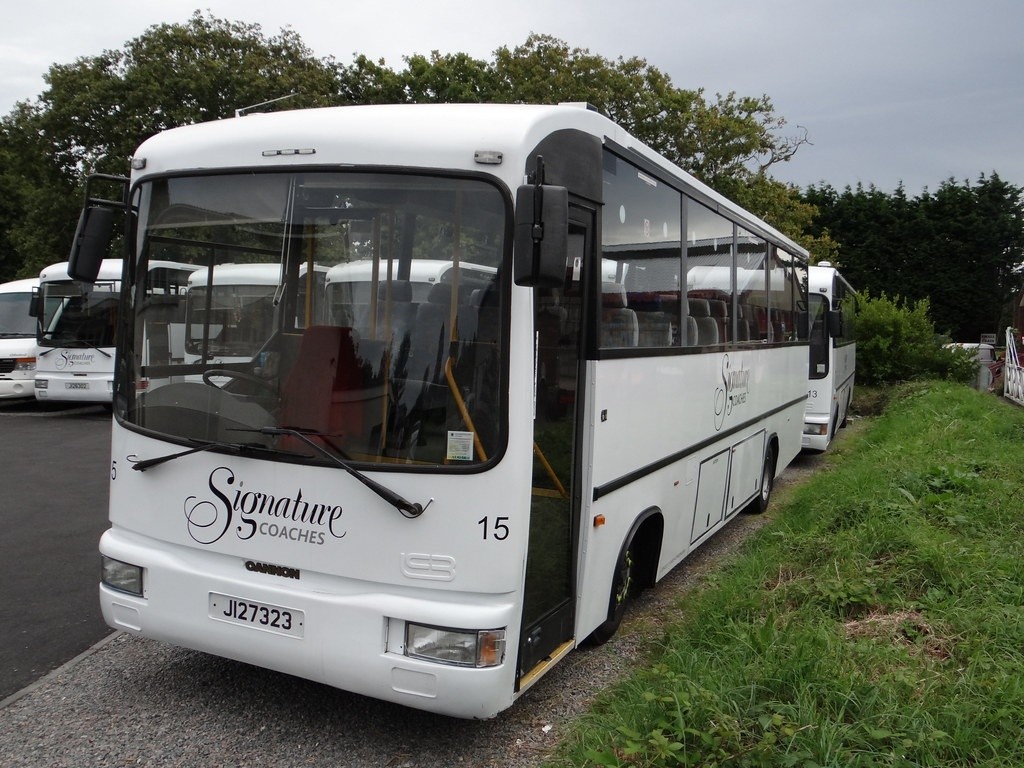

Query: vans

[946,343,997,374]
[0,278,46,400]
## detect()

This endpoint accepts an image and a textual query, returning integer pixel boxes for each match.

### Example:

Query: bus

[686,260,861,452]
[65,100,809,720]
[28,257,207,410]
[182,263,325,390]
[323,257,499,346]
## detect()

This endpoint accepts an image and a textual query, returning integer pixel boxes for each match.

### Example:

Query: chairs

[223,269,805,458]
